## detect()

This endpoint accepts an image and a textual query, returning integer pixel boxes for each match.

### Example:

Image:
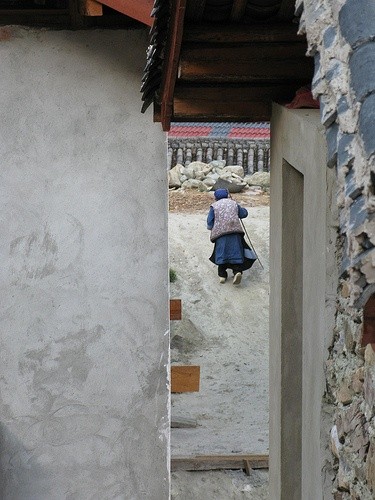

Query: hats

[214,188,228,200]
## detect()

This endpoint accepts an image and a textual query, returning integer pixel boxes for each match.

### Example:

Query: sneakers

[232,271,242,285]
[219,276,226,283]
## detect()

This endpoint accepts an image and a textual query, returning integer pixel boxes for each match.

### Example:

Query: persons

[206,188,248,284]
